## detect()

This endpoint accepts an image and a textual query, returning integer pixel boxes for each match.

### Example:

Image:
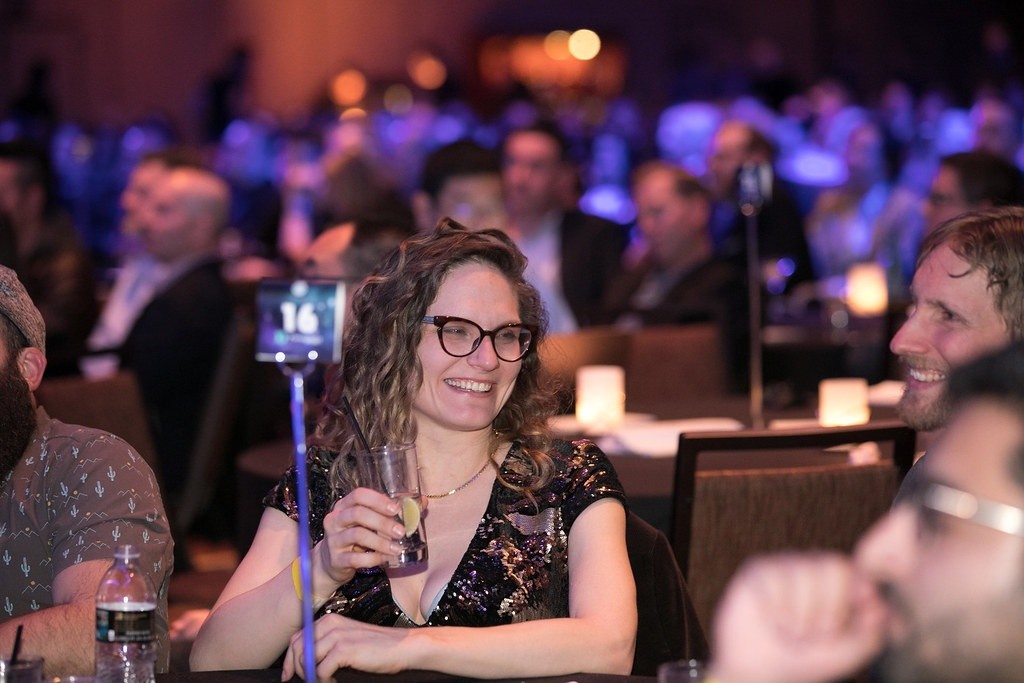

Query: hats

[0,264,46,355]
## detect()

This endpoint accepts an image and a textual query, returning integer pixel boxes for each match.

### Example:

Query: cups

[355,443,430,568]
[657,659,706,683]
[0,654,45,683]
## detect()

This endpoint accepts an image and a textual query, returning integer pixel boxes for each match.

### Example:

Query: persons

[0,265,173,682]
[703,341,1023,683]
[190,218,637,675]
[0,44,1023,566]
[891,210,1024,428]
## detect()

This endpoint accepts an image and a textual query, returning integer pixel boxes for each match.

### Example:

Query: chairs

[668,417,918,663]
[175,322,293,535]
[40,372,156,473]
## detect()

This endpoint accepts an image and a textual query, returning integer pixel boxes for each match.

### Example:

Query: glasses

[421,315,539,362]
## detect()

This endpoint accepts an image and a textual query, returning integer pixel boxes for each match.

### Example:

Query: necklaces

[419,427,500,497]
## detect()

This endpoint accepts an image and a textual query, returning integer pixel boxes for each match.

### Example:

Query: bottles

[92,544,157,683]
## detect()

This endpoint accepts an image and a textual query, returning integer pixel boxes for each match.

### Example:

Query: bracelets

[292,557,336,608]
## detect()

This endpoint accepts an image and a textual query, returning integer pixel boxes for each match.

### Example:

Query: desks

[551,410,893,499]
